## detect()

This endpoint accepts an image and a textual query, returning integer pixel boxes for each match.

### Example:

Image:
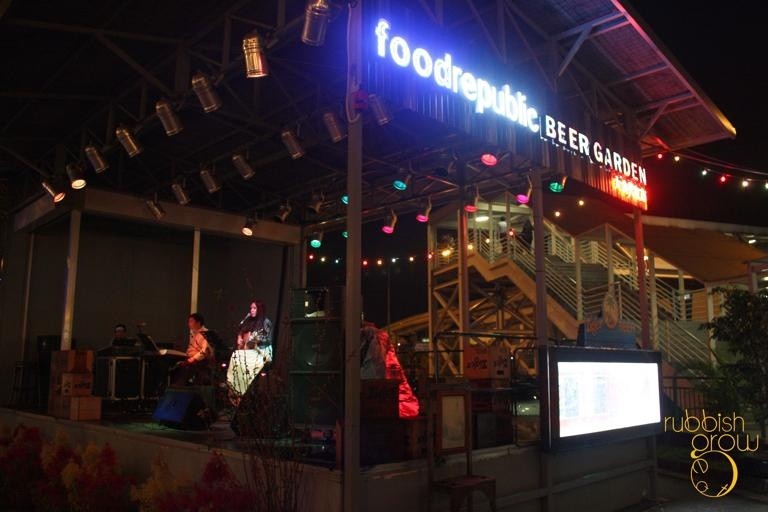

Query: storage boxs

[47,350,171,422]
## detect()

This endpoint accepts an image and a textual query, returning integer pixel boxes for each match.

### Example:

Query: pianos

[143,347,188,362]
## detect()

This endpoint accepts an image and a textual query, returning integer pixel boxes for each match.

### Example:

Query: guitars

[237,328,267,350]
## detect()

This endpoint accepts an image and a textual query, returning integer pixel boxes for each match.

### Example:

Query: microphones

[136,322,147,328]
[240,312,251,325]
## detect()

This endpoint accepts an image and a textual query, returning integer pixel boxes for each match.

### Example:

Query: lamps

[40,0,568,247]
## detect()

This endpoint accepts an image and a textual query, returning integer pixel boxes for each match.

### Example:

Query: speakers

[288,317,344,430]
[32,335,62,411]
[151,387,219,431]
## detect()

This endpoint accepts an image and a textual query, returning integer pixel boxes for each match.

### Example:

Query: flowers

[0,423,258,512]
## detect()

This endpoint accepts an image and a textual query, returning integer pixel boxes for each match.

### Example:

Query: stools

[7,361,39,410]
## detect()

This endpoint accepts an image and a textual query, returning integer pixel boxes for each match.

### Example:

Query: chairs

[426,383,496,512]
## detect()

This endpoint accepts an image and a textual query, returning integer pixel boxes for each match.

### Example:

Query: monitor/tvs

[539,344,665,453]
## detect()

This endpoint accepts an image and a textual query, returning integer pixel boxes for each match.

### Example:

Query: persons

[520,219,535,252]
[441,235,457,265]
[92,324,129,398]
[226,297,274,407]
[177,313,216,386]
[496,216,507,254]
